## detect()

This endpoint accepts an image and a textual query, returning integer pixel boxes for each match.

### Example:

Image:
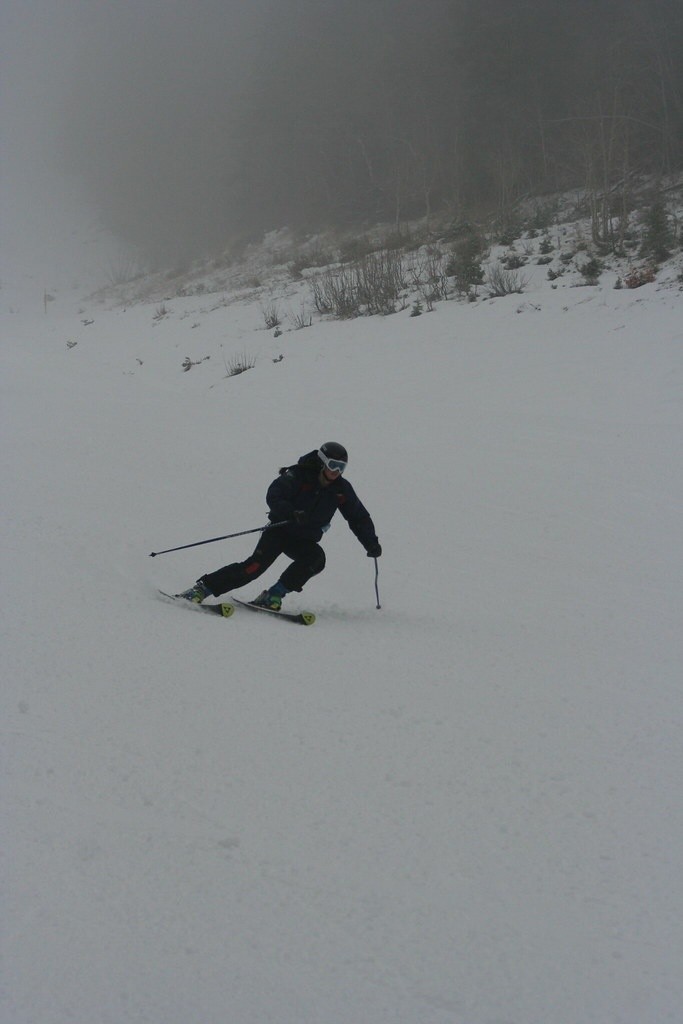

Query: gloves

[367,543,381,557]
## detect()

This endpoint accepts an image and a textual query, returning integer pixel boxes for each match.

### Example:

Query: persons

[172,441,383,612]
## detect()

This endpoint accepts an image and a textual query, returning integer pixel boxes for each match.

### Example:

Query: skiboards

[156,587,316,626]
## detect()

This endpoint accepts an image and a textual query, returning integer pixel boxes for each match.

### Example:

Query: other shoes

[180,582,213,604]
[254,590,282,611]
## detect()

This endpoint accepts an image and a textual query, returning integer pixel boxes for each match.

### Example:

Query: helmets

[318,442,348,472]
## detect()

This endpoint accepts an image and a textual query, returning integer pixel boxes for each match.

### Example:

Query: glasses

[327,459,347,473]
